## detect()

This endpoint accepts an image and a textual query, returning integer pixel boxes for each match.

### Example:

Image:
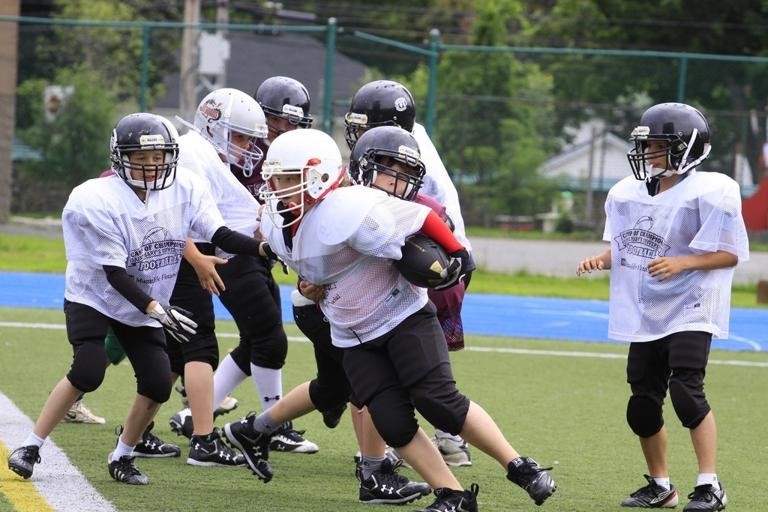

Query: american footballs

[391,235,451,288]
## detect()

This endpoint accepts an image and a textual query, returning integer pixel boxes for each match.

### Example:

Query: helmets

[626,102,712,184]
[345,80,426,201]
[110,113,179,191]
[193,88,269,178]
[254,76,313,148]
[259,130,346,230]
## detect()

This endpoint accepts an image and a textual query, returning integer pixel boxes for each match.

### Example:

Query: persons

[6,112,288,487]
[573,103,748,512]
[344,79,476,467]
[223,125,455,506]
[168,79,321,454]
[258,128,556,511]
[56,331,239,424]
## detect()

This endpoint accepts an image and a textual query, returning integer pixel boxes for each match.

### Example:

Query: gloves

[262,242,289,275]
[148,301,198,343]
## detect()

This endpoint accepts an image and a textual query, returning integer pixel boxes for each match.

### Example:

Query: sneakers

[115,421,181,458]
[683,481,727,512]
[270,419,319,454]
[620,474,679,508]
[8,445,41,478]
[353,444,431,505]
[414,483,479,512]
[108,451,150,485]
[506,456,557,506]
[429,433,472,467]
[59,398,106,424]
[169,386,274,484]
